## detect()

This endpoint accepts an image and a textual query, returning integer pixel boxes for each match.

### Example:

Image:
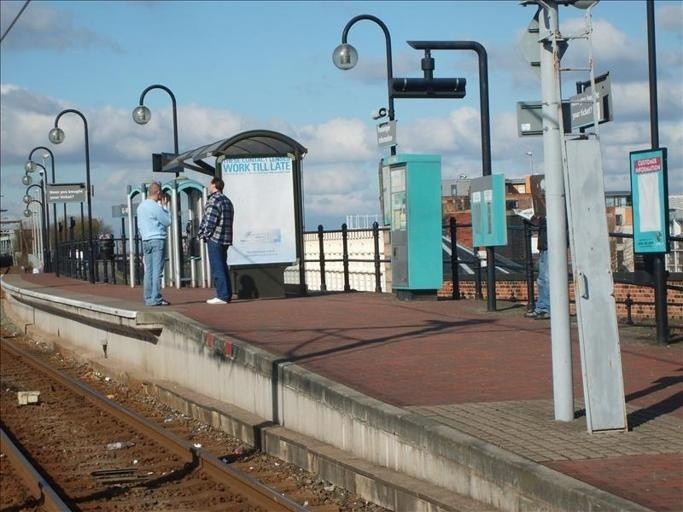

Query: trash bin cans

[97,233,114,260]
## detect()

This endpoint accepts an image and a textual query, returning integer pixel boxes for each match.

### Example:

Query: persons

[137,182,172,307]
[197,177,235,304]
[521,188,570,320]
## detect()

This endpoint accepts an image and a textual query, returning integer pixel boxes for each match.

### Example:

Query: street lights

[132,86,186,287]
[23,147,60,275]
[525,150,534,175]
[49,109,94,283]
[333,15,398,156]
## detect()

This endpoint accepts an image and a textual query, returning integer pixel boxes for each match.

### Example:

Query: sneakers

[206,297,229,305]
[144,299,171,306]
